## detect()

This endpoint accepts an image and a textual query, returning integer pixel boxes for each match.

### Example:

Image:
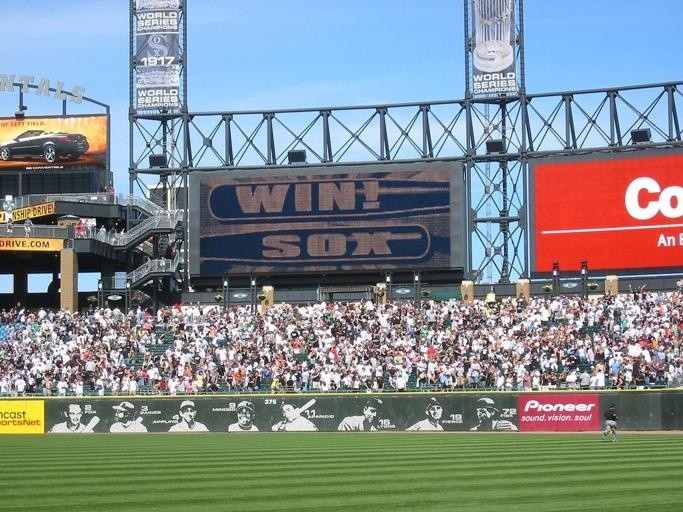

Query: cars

[0,129,89,163]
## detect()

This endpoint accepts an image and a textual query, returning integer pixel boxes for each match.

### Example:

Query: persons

[407,396,444,430]
[108,401,148,431]
[48,402,100,432]
[166,401,209,431]
[74,219,123,245]
[337,397,383,431]
[165,244,173,258]
[227,400,258,430]
[23,216,33,237]
[146,255,167,272]
[601,402,618,442]
[271,400,318,431]
[107,185,114,204]
[5,217,14,236]
[469,397,518,430]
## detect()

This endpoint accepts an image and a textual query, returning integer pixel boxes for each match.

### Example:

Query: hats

[477,398,500,414]
[112,401,134,412]
[425,397,440,411]
[180,401,195,410]
[364,399,383,410]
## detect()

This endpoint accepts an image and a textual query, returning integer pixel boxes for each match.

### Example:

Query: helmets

[235,400,255,417]
[279,401,297,410]
